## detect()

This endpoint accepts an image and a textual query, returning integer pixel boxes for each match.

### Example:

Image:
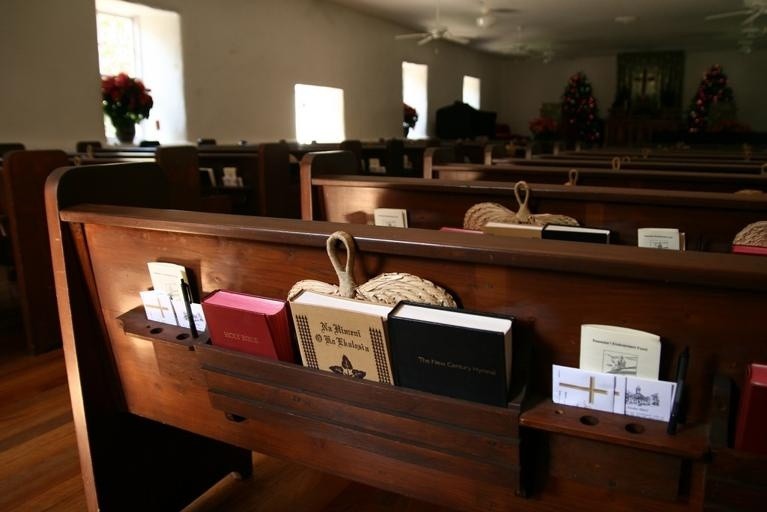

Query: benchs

[0,135,767,356]
[38,156,765,511]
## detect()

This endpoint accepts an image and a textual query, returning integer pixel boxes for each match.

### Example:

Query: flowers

[528,116,559,135]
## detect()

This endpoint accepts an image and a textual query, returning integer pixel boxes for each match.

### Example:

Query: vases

[113,118,138,146]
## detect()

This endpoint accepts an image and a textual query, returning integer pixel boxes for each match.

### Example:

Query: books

[439,227,483,235]
[483,221,543,240]
[541,222,613,244]
[200,289,296,363]
[287,287,394,387]
[387,300,515,408]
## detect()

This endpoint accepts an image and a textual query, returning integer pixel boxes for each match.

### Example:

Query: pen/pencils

[181,279,198,338]
[696,239,701,251]
[667,346,689,435]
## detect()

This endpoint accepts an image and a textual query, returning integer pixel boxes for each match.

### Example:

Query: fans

[395,0,483,47]
[704,1,767,55]
[100,73,154,129]
